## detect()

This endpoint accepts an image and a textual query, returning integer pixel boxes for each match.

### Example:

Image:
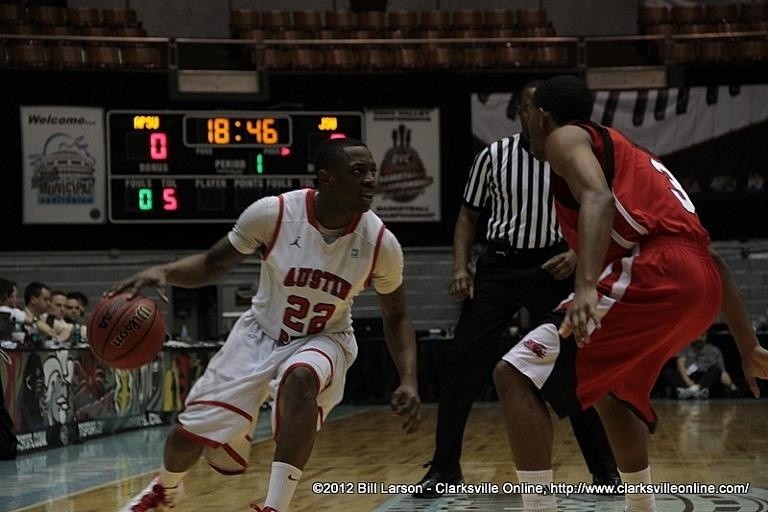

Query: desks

[340,316,768,405]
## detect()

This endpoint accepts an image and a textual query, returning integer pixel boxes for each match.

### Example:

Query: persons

[413,79,622,498]
[0,280,90,345]
[676,329,736,401]
[491,75,768,512]
[102,139,422,512]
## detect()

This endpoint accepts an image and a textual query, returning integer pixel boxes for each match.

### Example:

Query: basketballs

[86,293,166,370]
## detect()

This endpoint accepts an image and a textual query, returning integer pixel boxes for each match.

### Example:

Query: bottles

[30,317,43,348]
[180,324,187,337]
[10,320,25,345]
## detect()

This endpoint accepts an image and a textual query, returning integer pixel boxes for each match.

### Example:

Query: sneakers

[120,475,185,512]
[677,387,708,400]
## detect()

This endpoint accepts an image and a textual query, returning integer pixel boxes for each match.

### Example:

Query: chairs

[227,1,561,65]
[636,1,768,69]
[1,1,161,70]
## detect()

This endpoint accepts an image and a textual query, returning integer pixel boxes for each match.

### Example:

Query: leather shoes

[412,461,463,498]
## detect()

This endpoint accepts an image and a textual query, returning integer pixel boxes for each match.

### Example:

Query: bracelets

[728,382,733,387]
